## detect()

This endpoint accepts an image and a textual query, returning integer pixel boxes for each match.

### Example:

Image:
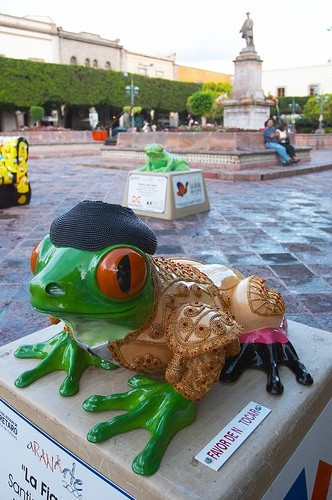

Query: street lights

[123,72,134,128]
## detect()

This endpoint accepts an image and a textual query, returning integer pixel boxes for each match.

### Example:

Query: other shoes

[283,157,300,165]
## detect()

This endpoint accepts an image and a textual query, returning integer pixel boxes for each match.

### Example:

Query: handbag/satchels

[285,138,290,144]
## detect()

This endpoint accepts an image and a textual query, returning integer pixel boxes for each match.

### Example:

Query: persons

[18,111,197,140]
[263,118,293,166]
[238,12,254,49]
[275,122,300,165]
